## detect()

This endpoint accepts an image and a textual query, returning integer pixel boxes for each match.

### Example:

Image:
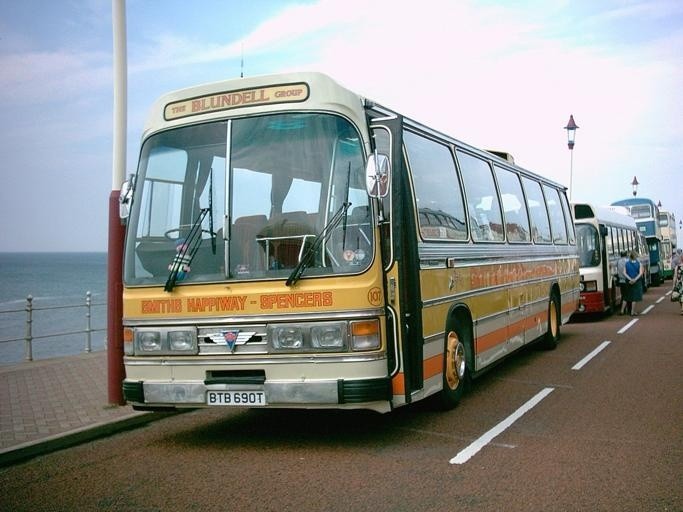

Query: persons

[615,251,632,315]
[617,251,644,316]
[670,248,682,270]
[670,255,683,314]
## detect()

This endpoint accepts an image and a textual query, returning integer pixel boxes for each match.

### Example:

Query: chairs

[232,206,372,269]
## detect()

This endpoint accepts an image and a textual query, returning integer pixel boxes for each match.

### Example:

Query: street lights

[564,115,579,198]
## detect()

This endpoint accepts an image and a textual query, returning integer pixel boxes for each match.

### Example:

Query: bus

[610,197,662,286]
[563,203,642,318]
[116,72,582,416]
[657,211,678,281]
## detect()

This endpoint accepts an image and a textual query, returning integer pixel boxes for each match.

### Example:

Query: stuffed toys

[167,238,192,282]
